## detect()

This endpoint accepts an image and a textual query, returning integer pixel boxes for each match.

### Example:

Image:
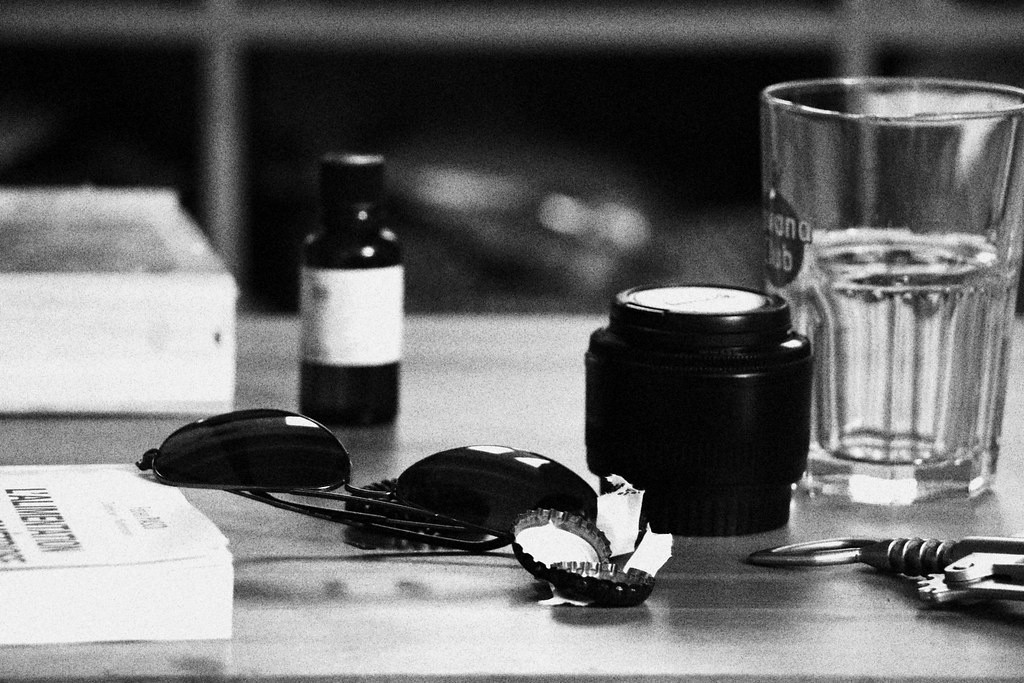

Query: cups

[764,79,1024,508]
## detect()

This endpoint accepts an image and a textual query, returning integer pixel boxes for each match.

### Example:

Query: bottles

[298,155,405,426]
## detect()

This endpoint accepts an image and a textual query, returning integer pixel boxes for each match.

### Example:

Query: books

[0,464,235,644]
[0,189,237,418]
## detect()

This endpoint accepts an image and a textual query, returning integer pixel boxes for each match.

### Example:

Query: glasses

[136,408,600,552]
